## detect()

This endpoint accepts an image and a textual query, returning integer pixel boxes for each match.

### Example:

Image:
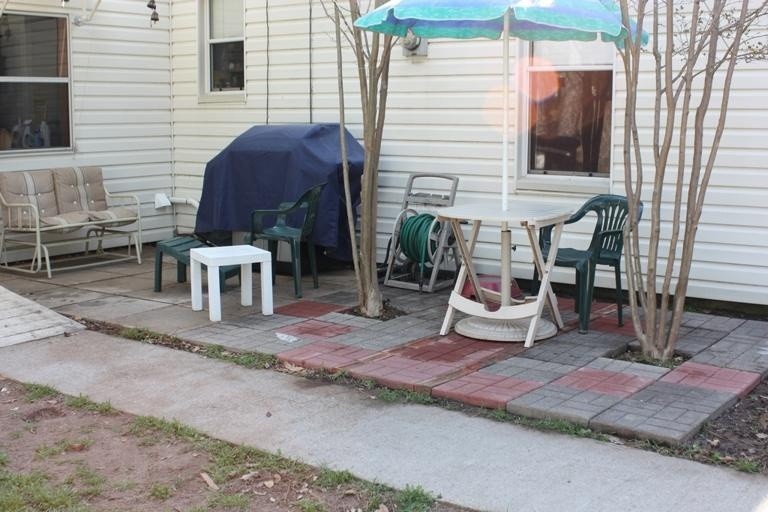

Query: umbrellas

[352,0,650,308]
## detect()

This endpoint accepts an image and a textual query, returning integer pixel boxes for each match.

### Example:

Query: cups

[534,152,545,170]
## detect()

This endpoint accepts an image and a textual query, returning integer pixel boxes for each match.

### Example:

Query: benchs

[0,164,146,280]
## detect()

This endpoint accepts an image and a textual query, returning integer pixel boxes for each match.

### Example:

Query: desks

[430,200,577,350]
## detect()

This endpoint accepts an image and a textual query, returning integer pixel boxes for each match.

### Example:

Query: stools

[188,243,276,324]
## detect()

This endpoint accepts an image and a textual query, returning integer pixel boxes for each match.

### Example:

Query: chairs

[529,191,644,335]
[242,180,329,300]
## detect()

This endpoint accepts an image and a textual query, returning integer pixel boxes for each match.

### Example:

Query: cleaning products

[0,116,51,150]
